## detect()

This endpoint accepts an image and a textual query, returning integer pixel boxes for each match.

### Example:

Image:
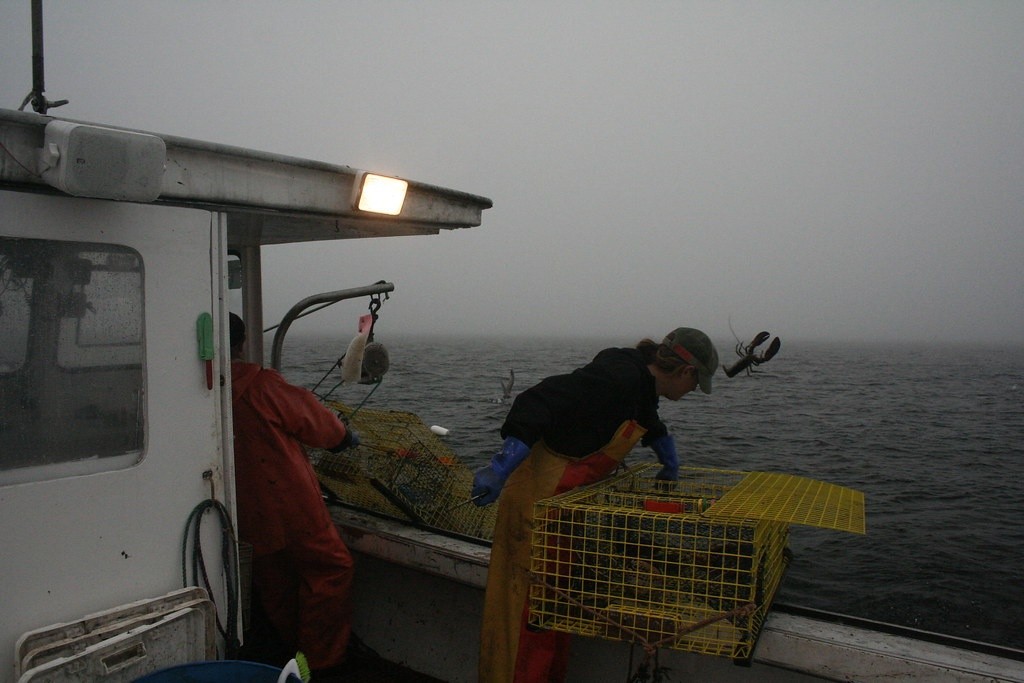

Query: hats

[664,327,719,394]
[229,311,245,347]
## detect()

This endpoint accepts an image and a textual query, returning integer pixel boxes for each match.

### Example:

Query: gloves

[325,425,360,454]
[470,435,530,507]
[649,435,679,492]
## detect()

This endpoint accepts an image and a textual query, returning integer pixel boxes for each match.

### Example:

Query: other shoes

[310,655,364,679]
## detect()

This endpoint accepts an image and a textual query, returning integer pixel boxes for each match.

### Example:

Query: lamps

[348,170,409,218]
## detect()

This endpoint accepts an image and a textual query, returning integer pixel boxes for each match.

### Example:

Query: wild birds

[500,369,514,399]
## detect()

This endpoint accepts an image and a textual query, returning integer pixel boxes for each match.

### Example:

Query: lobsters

[723,331,780,377]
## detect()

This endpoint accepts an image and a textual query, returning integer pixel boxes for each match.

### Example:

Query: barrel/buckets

[130,660,298,683]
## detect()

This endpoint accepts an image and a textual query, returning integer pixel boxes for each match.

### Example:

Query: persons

[228,313,358,683]
[471,327,718,683]
